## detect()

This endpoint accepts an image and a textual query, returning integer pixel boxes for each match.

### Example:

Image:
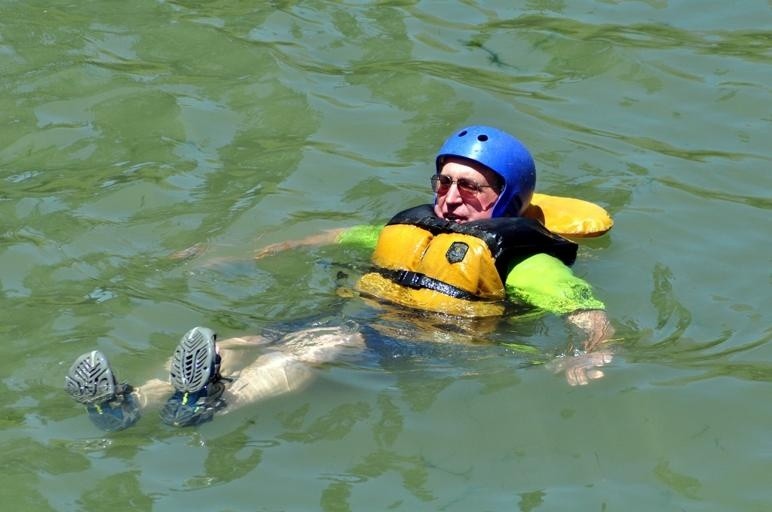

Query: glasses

[430,175,504,198]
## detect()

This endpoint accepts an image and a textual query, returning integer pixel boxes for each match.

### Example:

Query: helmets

[434,126,536,218]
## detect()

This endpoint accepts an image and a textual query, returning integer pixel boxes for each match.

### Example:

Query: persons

[64,124,615,434]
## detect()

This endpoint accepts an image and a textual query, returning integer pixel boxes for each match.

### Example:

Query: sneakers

[64,351,141,433]
[158,327,233,426]
